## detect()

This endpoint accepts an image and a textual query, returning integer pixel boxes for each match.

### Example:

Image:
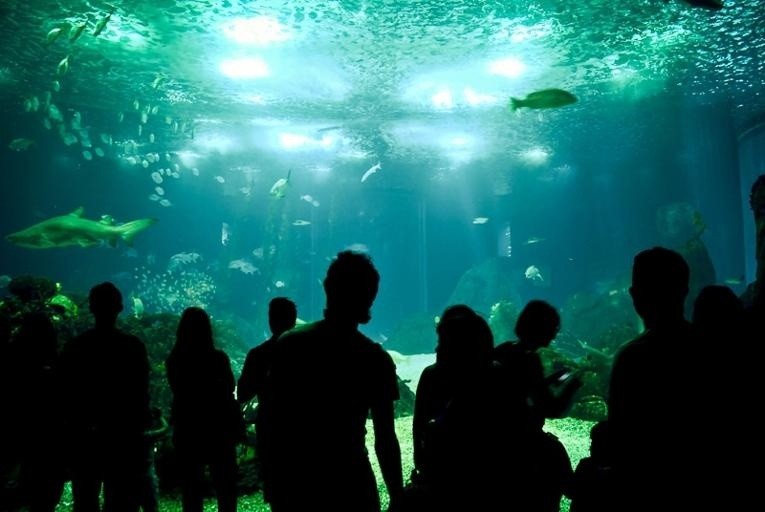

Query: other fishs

[508,88,579,110]
[7,12,383,208]
[4,205,156,250]
[523,264,544,282]
[219,218,311,274]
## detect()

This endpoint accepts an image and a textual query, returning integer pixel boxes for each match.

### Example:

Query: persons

[607,246,715,426]
[495,299,582,416]
[413,305,503,512]
[269,250,403,511]
[165,307,243,511]
[65,283,150,510]
[520,403,574,512]
[240,298,296,504]
